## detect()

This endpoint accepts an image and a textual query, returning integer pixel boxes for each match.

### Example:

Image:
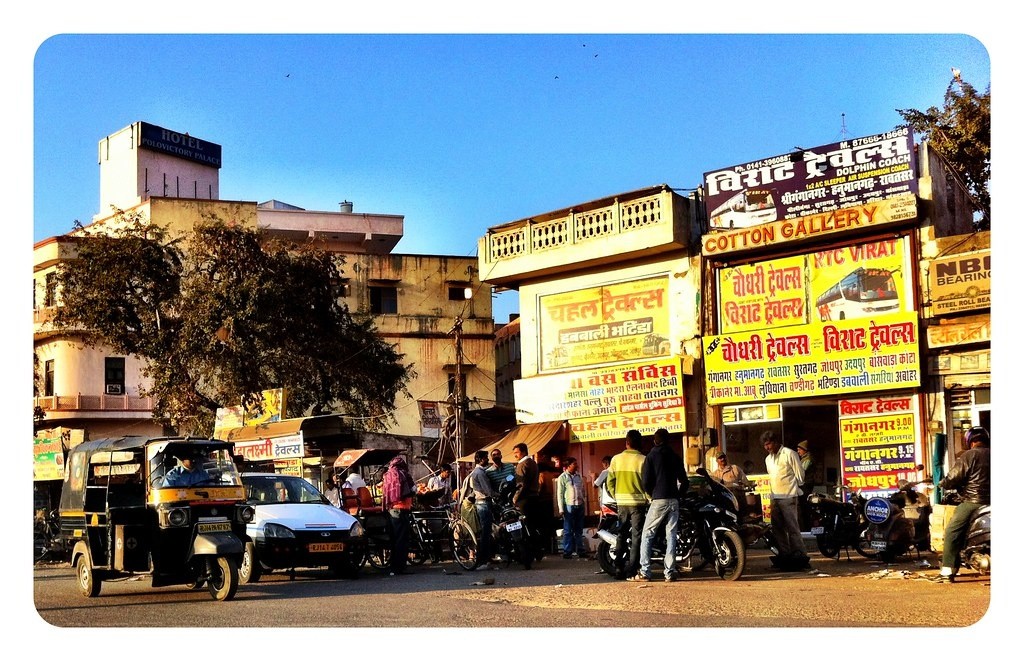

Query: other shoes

[665,577,677,582]
[579,554,589,558]
[476,565,490,570]
[626,574,649,582]
[393,566,417,574]
[563,555,574,559]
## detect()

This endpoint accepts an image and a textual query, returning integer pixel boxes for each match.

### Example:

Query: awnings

[457,420,565,463]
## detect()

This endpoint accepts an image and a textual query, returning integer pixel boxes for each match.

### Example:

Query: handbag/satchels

[783,550,810,572]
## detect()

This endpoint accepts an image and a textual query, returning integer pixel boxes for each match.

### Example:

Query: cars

[236,473,363,583]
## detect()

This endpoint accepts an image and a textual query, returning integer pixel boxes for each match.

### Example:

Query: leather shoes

[927,574,954,583]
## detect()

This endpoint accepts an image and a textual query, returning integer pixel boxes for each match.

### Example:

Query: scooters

[878,465,935,561]
[960,506,991,576]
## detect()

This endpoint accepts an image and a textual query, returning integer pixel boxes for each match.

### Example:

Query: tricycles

[334,448,479,570]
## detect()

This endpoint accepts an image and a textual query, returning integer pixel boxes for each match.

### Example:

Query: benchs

[108,488,153,524]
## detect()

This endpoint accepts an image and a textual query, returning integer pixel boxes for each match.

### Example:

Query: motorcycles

[806,485,884,560]
[60,435,255,602]
[483,476,532,567]
[594,468,745,583]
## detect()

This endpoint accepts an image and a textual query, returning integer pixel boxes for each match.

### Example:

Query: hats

[716,452,726,459]
[798,440,807,450]
[440,464,452,471]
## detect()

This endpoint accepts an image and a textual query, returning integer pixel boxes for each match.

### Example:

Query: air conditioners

[106,384,121,395]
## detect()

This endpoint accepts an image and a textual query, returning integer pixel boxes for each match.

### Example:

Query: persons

[383,443,546,563]
[161,455,212,486]
[711,450,752,486]
[607,427,690,582]
[557,456,611,560]
[760,430,815,570]
[926,426,990,584]
[324,478,339,509]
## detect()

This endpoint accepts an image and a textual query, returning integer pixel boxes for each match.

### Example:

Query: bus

[710,188,778,230]
[816,265,903,321]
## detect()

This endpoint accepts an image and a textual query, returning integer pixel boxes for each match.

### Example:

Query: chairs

[264,486,279,501]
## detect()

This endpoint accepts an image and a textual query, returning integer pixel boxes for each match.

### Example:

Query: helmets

[966,426,989,450]
